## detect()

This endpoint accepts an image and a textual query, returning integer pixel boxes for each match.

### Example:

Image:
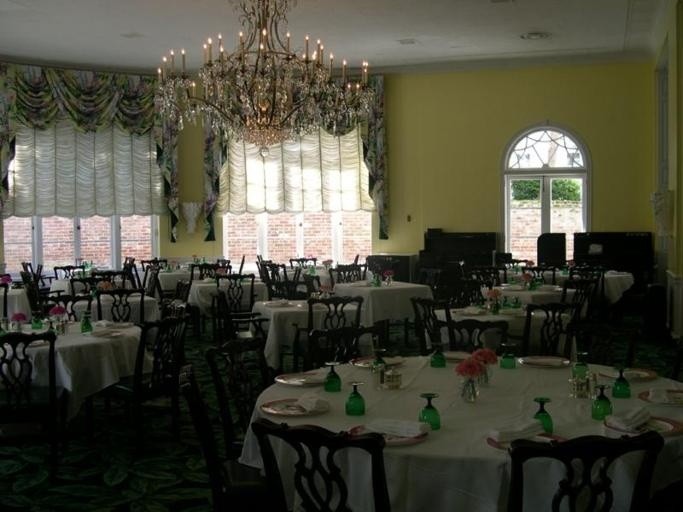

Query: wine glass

[562,264,568,275]
[499,344,516,369]
[474,301,482,309]
[492,303,498,313]
[373,271,381,288]
[311,266,315,274]
[611,366,631,399]
[571,351,589,379]
[591,385,613,421]
[429,342,446,368]
[371,348,387,365]
[81,315,91,332]
[29,310,41,329]
[511,296,521,308]
[344,381,365,416]
[502,295,510,308]
[324,360,342,393]
[532,395,553,435]
[417,391,441,430]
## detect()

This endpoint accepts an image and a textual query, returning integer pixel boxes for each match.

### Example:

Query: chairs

[292,295,364,372]
[411,297,456,352]
[34,264,47,286]
[521,302,581,363]
[191,263,219,281]
[141,257,168,272]
[216,274,258,331]
[417,268,443,299]
[104,318,181,415]
[181,383,263,512]
[289,258,317,270]
[260,263,288,297]
[21,262,57,285]
[158,280,192,318]
[216,258,232,274]
[303,274,320,297]
[475,265,508,284]
[570,267,604,298]
[91,271,125,289]
[210,293,270,352]
[0,330,69,449]
[20,271,65,297]
[337,264,368,283]
[70,277,111,296]
[123,263,141,289]
[501,259,528,271]
[256,261,272,276]
[96,289,145,323]
[257,255,262,261]
[217,290,233,329]
[251,417,393,512]
[121,257,135,280]
[238,255,245,275]
[22,281,65,317]
[571,271,600,280]
[143,265,161,297]
[522,266,555,286]
[562,280,598,305]
[303,325,363,370]
[289,267,301,299]
[46,294,93,322]
[329,267,361,288]
[202,351,276,459]
[54,265,86,280]
[0,283,9,317]
[507,431,664,512]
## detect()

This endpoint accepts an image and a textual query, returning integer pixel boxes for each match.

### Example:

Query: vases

[192,258,196,263]
[489,298,501,314]
[478,365,490,387]
[528,280,536,288]
[172,266,175,271]
[460,377,479,403]
[384,278,392,286]
[55,323,62,335]
[14,323,21,332]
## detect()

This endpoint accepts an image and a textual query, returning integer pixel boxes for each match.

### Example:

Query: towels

[649,386,670,403]
[463,306,487,314]
[365,416,432,438]
[600,367,641,380]
[605,407,653,430]
[94,320,113,326]
[87,328,110,337]
[487,413,546,441]
[302,369,330,383]
[297,393,331,412]
[265,299,291,306]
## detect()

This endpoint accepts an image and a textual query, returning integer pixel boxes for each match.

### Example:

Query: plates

[638,385,683,407]
[273,372,326,387]
[486,428,569,452]
[544,284,560,291]
[115,322,134,328]
[348,355,408,368]
[444,358,467,363]
[260,396,321,418]
[602,410,682,440]
[517,355,571,368]
[347,422,426,448]
[502,285,525,291]
[30,339,50,347]
[93,329,123,337]
[599,365,658,382]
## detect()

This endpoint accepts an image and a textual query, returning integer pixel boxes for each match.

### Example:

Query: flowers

[383,271,394,277]
[48,306,66,323]
[470,349,499,366]
[323,260,328,267]
[191,254,198,258]
[327,259,333,265]
[523,272,532,279]
[568,260,575,266]
[527,261,534,266]
[455,358,483,378]
[10,313,26,324]
[0,276,13,285]
[171,260,176,266]
[487,290,502,299]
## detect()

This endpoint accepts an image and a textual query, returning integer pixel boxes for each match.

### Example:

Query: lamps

[153,0,376,163]
